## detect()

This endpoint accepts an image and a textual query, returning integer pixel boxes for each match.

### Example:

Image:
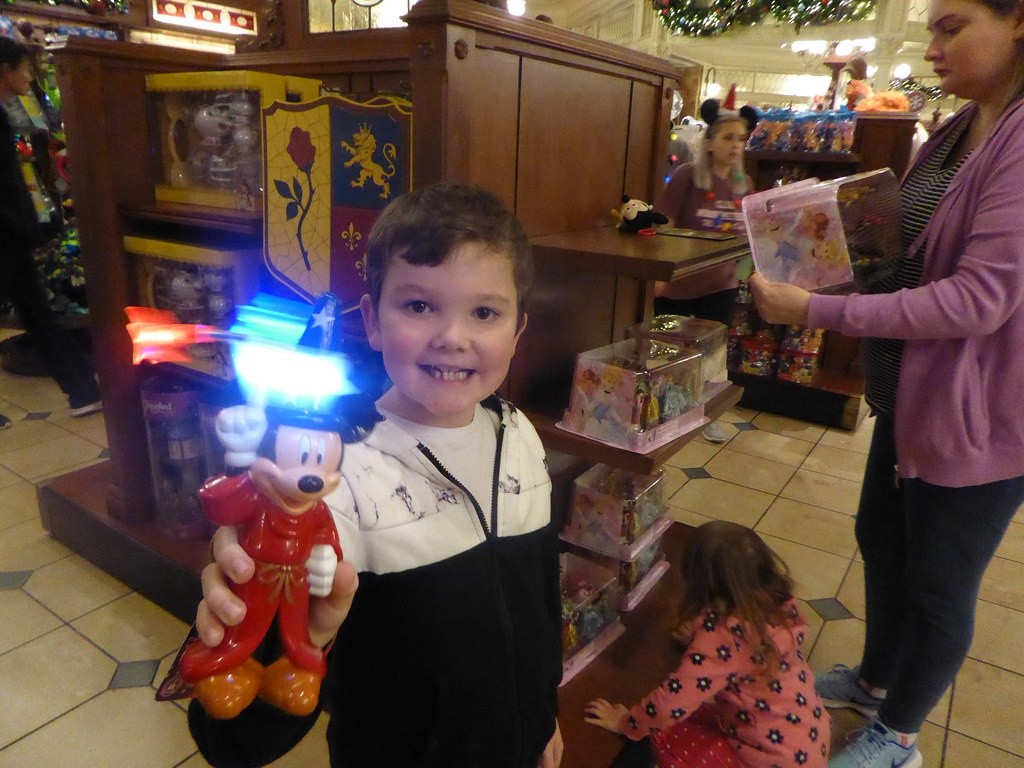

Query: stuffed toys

[613,195,669,235]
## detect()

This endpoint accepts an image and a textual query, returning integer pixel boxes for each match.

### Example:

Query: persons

[652,99,759,443]
[582,520,833,768]
[185,182,564,768]
[746,0,1024,768]
[0,35,104,431]
[631,374,687,432]
[564,590,609,650]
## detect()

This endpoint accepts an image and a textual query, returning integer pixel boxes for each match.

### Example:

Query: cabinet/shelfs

[725,111,920,433]
[32,0,751,768]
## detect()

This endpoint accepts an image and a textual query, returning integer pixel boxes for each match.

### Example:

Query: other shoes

[702,422,725,442]
[68,384,102,417]
[0,414,11,431]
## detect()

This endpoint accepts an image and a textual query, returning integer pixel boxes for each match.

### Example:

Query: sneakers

[827,717,923,768]
[812,664,884,717]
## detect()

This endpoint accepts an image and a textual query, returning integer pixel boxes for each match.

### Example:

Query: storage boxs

[740,166,907,298]
[551,313,735,688]
[123,70,321,548]
